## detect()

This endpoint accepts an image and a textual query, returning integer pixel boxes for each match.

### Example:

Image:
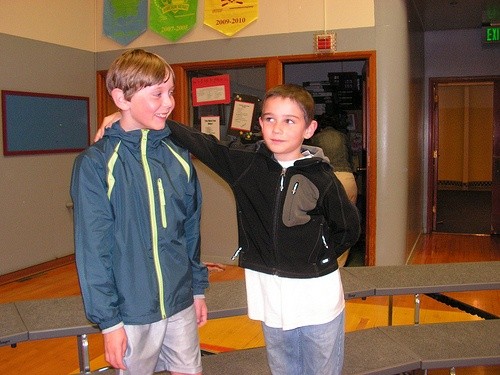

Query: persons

[68,48,361,375]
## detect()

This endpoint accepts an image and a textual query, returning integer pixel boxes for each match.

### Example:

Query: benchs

[0,261,500,375]
